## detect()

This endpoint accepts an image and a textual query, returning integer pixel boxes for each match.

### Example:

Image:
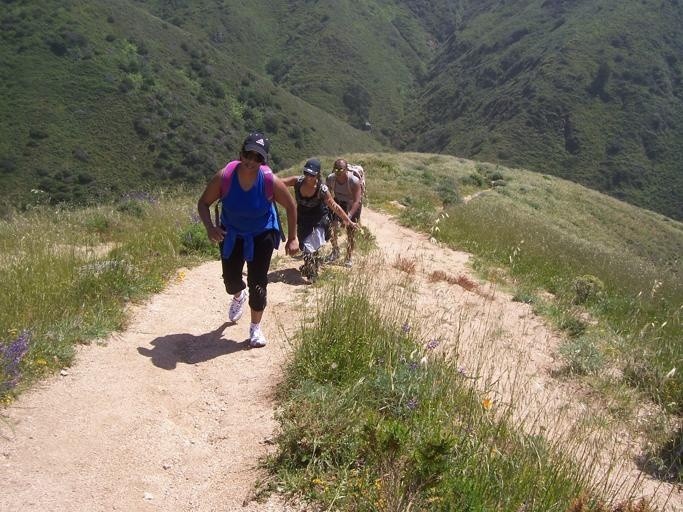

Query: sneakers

[249,323,266,347]
[229,289,249,322]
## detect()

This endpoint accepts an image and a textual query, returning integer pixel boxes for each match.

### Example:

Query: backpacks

[332,164,365,196]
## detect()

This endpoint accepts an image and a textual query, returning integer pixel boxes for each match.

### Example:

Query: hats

[243,132,270,159]
[304,159,320,176]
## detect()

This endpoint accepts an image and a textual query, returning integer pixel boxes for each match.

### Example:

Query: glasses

[243,152,263,162]
[334,169,344,172]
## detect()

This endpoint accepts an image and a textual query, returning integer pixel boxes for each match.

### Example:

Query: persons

[280,159,364,280]
[196,132,300,348]
[322,157,362,268]
